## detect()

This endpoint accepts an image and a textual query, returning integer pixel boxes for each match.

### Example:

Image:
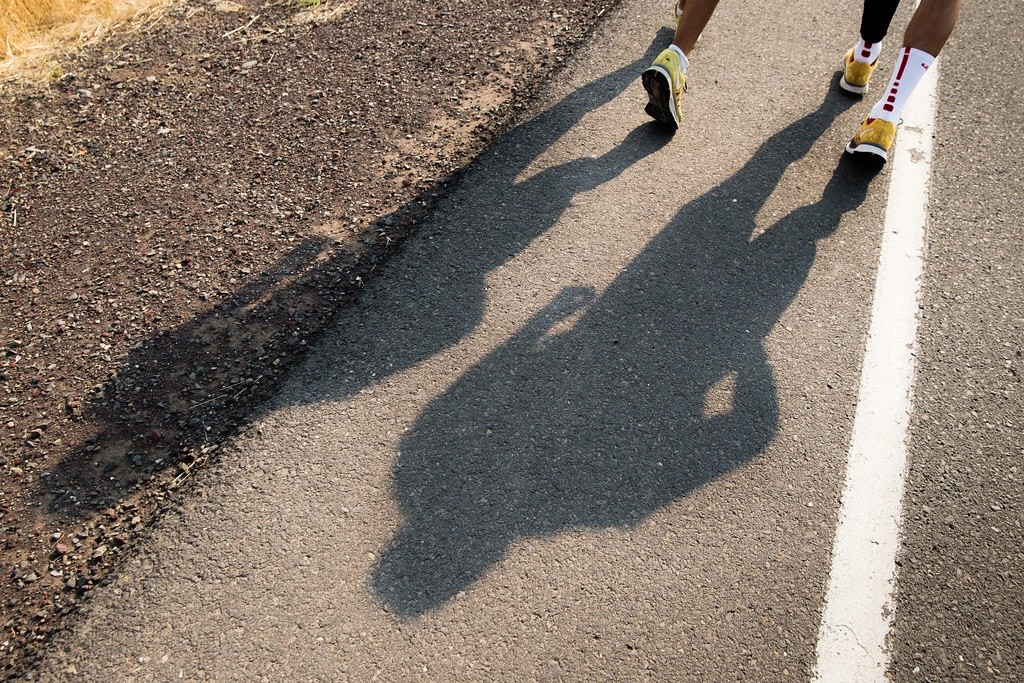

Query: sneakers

[840,46,880,94]
[846,116,904,164]
[674,1,699,41]
[641,49,688,129]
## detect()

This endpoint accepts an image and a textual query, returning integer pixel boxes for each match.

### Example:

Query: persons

[640,0,719,130]
[839,0,962,164]
